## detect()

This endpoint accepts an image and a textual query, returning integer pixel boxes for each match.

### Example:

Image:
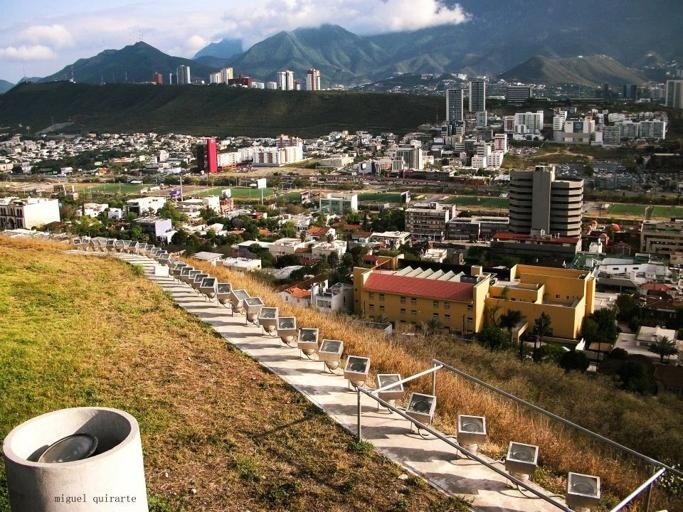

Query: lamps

[37,236,602,512]
